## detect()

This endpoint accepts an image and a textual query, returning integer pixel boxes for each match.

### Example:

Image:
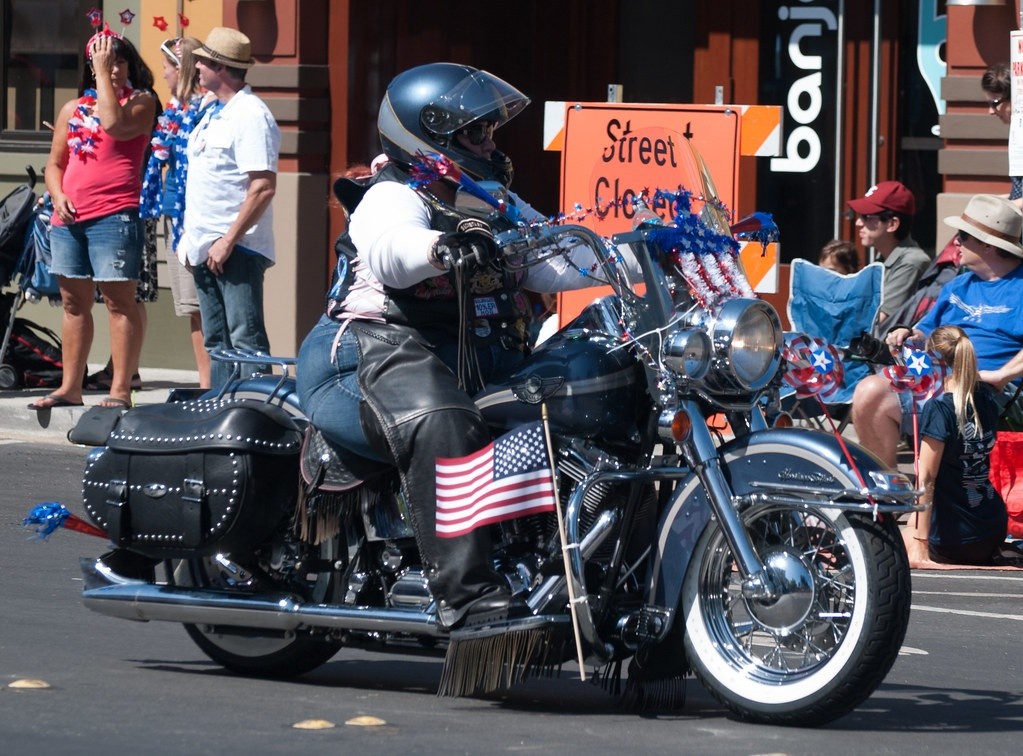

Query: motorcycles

[76,130,926,721]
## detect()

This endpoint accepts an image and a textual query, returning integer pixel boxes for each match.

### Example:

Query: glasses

[456,124,493,145]
[861,214,875,221]
[958,229,986,244]
[988,97,1009,108]
[161,38,180,65]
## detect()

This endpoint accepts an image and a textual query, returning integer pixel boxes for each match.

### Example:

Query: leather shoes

[436,593,531,629]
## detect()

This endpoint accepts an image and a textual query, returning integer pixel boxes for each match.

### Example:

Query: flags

[435,417,558,539]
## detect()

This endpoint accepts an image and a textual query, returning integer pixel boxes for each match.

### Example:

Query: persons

[151,26,280,389]
[851,194,1023,565]
[817,181,931,325]
[26,30,155,410]
[295,62,644,615]
[981,64,1023,207]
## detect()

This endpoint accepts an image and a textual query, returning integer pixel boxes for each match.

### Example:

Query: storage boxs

[63,399,304,557]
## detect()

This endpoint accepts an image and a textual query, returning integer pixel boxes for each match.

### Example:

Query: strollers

[0,164,53,390]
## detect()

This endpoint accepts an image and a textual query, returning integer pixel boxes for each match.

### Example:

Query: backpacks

[1,317,88,388]
[0,183,37,288]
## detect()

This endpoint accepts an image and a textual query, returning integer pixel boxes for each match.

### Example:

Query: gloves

[431,231,504,274]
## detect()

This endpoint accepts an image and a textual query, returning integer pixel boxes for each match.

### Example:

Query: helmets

[376,62,514,191]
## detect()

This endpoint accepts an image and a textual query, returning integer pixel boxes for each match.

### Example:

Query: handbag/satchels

[989,432,1023,539]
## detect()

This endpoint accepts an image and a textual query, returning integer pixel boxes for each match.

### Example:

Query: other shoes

[88,372,142,391]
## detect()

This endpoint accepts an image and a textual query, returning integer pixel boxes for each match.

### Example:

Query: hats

[191,27,256,69]
[942,194,1023,258]
[847,181,915,215]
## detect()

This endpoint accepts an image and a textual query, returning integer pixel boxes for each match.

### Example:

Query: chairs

[760,259,885,434]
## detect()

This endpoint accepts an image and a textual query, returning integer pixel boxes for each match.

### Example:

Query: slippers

[27,394,83,410]
[101,398,132,410]
